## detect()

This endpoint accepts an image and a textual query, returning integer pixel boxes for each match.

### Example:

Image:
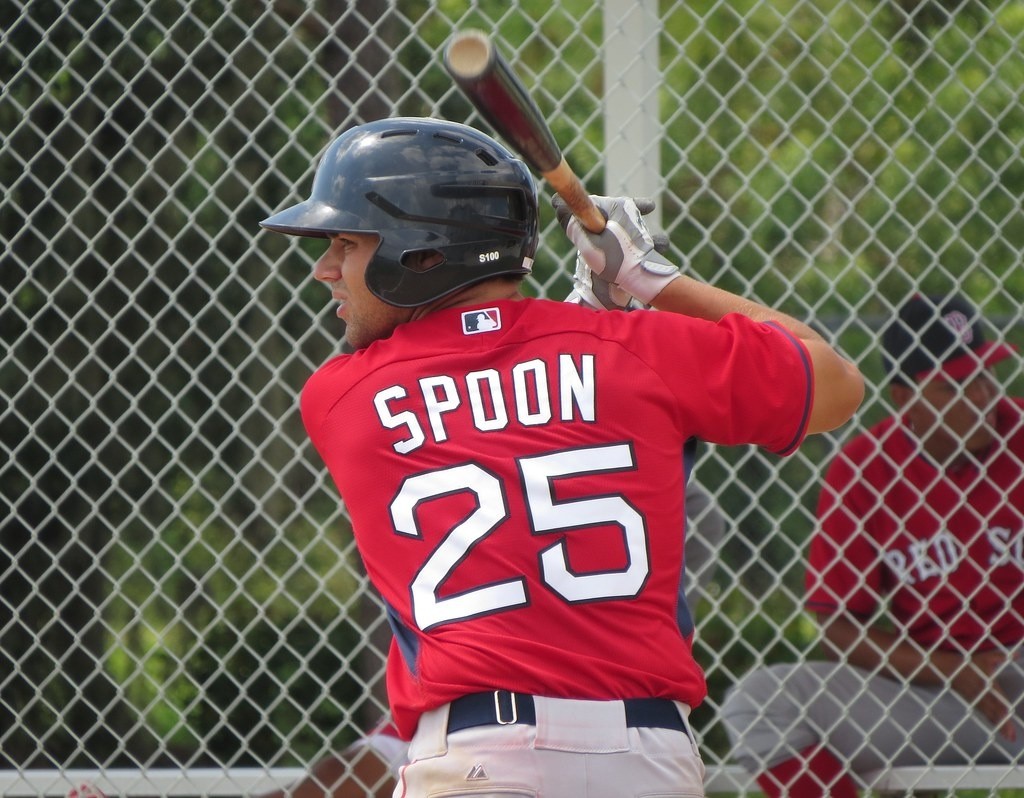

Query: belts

[447,690,690,736]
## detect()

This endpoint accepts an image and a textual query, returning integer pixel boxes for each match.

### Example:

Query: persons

[721,294,1024,798]
[258,117,865,798]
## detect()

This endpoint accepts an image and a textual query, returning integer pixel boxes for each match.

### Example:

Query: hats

[881,290,1020,381]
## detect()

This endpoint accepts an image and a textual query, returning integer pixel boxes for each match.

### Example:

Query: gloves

[550,192,682,312]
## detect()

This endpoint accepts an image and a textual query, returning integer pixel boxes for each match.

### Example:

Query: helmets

[258,117,539,309]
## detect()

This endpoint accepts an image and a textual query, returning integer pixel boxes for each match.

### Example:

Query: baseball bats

[441,30,607,234]
